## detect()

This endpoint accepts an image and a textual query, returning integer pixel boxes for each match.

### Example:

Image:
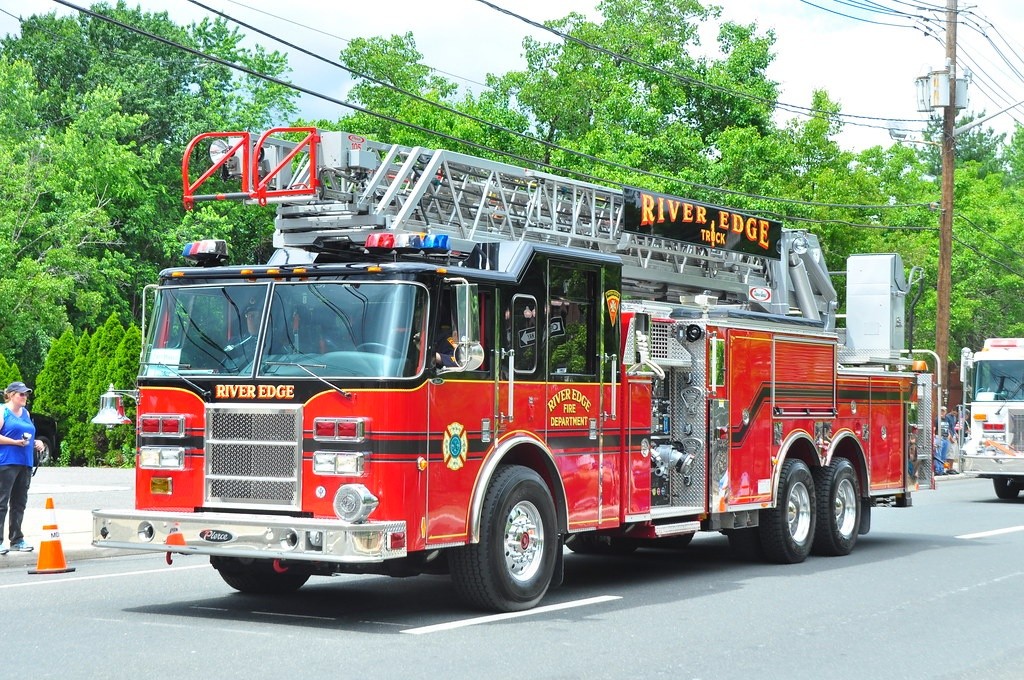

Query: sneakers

[10,542,34,552]
[0,544,9,554]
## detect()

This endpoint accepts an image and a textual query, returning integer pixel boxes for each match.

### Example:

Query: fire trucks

[91,127,943,614]
[959,338,1024,499]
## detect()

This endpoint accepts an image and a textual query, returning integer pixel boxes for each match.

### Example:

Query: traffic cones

[28,498,76,575]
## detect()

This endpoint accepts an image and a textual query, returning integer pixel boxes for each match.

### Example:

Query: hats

[245,295,264,311]
[941,406,947,411]
[6,382,32,394]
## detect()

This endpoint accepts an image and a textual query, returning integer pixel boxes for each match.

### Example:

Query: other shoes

[942,472,947,475]
[947,469,959,474]
[934,472,942,476]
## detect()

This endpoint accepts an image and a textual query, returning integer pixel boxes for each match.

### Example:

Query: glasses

[14,393,27,397]
[245,311,260,318]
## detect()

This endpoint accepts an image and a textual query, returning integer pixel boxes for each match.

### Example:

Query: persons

[908,405,970,484]
[0,382,45,555]
[225,298,460,368]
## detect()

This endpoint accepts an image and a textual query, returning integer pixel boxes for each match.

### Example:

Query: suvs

[0,390,57,466]
